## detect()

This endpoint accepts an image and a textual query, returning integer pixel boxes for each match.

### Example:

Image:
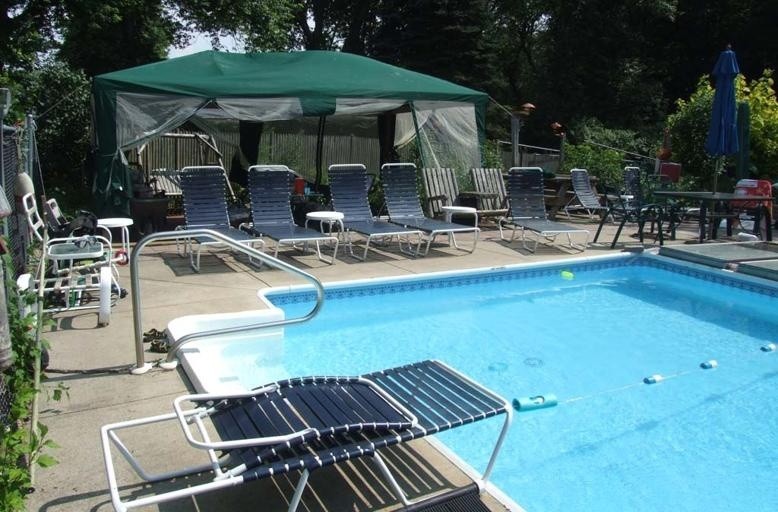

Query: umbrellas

[703,43,742,240]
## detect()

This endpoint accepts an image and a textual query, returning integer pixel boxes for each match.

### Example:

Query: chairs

[100,358,515,512]
[22,191,134,328]
